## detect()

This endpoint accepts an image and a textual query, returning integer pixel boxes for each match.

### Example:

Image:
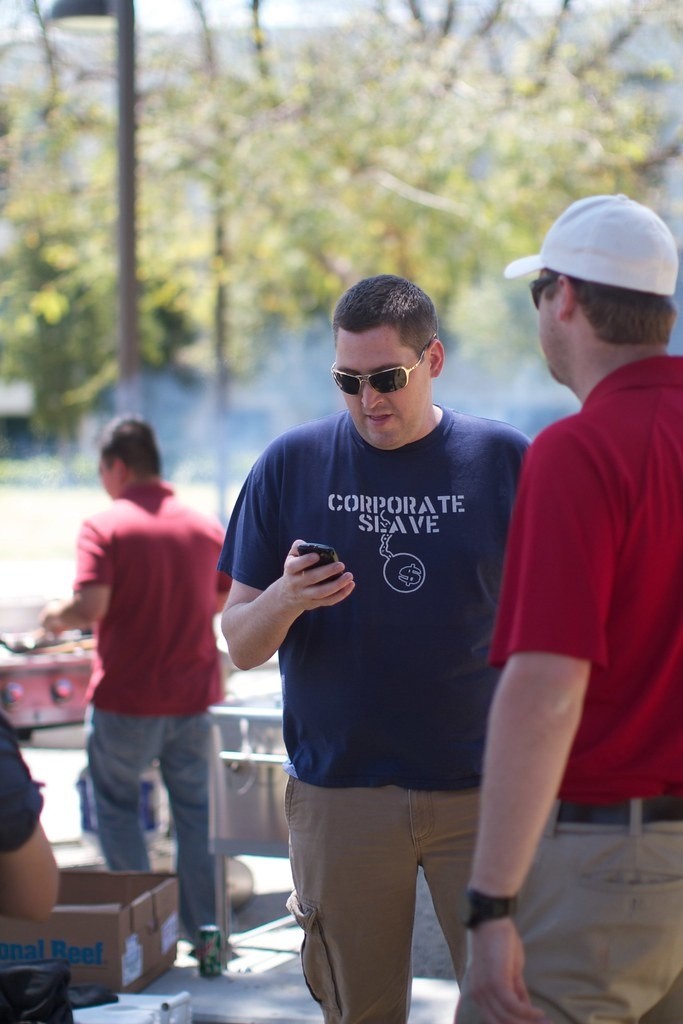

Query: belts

[558,797,683,826]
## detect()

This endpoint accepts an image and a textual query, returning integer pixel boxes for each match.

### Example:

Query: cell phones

[298,543,344,584]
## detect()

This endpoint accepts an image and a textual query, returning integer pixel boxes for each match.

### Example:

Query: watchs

[462,890,517,928]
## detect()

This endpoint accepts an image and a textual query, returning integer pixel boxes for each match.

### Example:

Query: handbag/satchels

[0,964,77,1024]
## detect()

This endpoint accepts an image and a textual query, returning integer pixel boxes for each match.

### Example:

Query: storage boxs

[1,868,179,996]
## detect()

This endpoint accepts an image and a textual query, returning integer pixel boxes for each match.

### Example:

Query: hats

[503,192,680,296]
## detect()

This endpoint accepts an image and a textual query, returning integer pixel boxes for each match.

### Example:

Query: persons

[39,415,232,953]
[455,196,683,1024]
[0,683,60,921]
[217,275,531,1024]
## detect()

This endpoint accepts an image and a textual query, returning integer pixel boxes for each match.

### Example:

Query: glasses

[529,278,557,309]
[330,332,441,397]
